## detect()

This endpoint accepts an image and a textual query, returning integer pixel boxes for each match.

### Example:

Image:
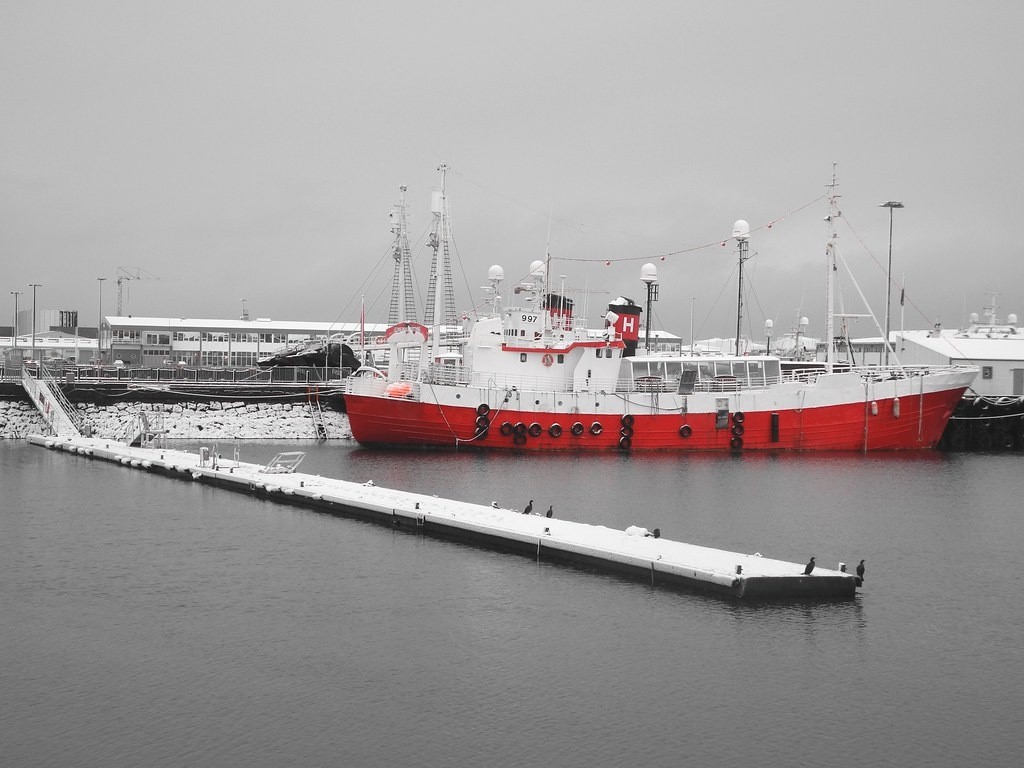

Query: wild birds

[801,557,815,575]
[654,529,660,538]
[856,559,865,580]
[522,500,535,515]
[546,505,553,518]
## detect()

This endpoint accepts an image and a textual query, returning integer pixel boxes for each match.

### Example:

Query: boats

[942,395,1024,450]
[343,164,978,450]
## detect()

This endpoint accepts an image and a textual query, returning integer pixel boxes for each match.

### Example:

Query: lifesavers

[500,421,543,437]
[679,423,692,438]
[571,421,584,435]
[618,414,634,449]
[977,433,1018,452]
[730,411,745,449]
[589,421,603,435]
[474,403,490,440]
[950,434,965,448]
[548,422,563,438]
[513,433,527,446]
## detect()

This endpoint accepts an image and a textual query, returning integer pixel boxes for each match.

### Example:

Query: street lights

[96,278,106,378]
[10,292,23,349]
[29,284,42,360]
